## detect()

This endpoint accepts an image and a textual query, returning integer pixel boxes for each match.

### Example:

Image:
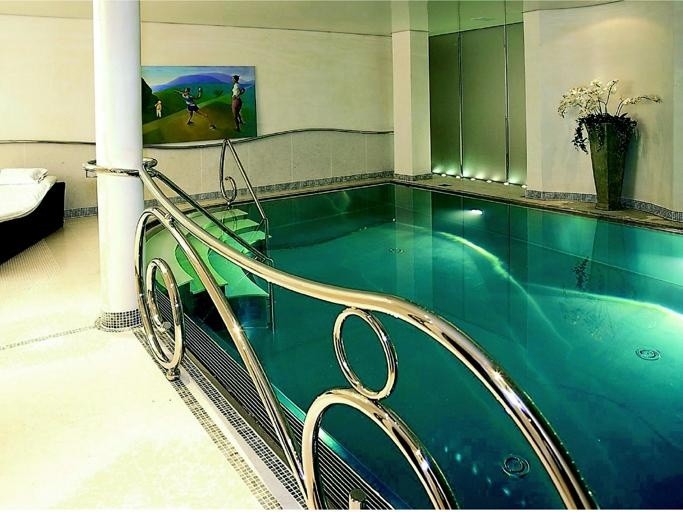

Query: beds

[0,168,65,265]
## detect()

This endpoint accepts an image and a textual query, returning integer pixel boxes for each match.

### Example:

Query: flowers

[556,78,665,116]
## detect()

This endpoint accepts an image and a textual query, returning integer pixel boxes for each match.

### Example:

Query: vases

[583,121,633,211]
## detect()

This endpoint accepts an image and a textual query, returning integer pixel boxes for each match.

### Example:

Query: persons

[180,86,216,130]
[154,101,162,118]
[230,75,245,133]
